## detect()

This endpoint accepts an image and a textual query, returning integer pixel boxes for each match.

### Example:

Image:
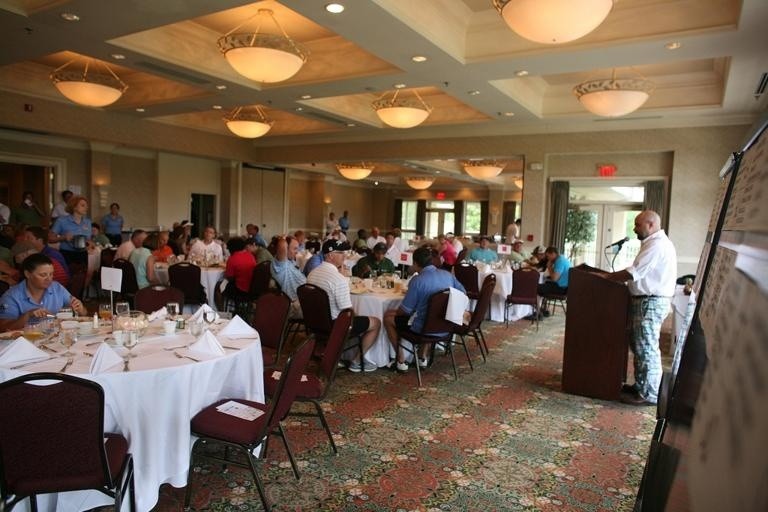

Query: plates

[76,330,98,335]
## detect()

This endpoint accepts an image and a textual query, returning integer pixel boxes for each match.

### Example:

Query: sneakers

[348,360,378,372]
[417,357,427,370]
[386,361,409,373]
[338,362,345,368]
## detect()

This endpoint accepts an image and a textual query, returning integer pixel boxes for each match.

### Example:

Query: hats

[182,221,194,227]
[334,225,341,231]
[322,238,351,254]
[374,242,388,251]
[531,245,546,254]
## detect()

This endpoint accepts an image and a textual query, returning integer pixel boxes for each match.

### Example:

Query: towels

[189,329,224,356]
[185,303,218,326]
[443,287,469,327]
[89,341,124,378]
[216,314,254,337]
[0,336,49,363]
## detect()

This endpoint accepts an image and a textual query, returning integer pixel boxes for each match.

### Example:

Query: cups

[167,303,179,318]
[189,321,201,342]
[60,321,74,356]
[115,304,129,316]
[57,307,73,322]
[77,321,92,333]
[24,323,41,344]
[395,271,401,281]
[203,305,215,331]
[121,330,138,359]
[369,270,377,284]
[176,318,185,329]
[99,306,113,330]
[165,320,176,335]
[113,331,126,346]
[41,318,54,345]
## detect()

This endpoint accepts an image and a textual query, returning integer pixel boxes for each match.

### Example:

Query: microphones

[606,236,629,248]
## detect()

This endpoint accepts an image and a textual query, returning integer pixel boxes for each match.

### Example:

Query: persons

[100,203,123,247]
[507,239,528,263]
[352,229,367,251]
[327,225,346,241]
[383,247,471,374]
[50,190,73,224]
[0,202,10,231]
[384,232,399,268]
[270,235,306,302]
[249,225,266,248]
[158,231,169,247]
[153,226,187,263]
[293,230,306,251]
[437,233,457,271]
[10,190,46,228]
[521,245,549,272]
[186,225,223,262]
[325,212,338,238]
[524,246,573,323]
[446,232,463,256]
[0,254,88,331]
[504,218,521,245]
[366,226,386,249]
[128,235,158,290]
[23,226,69,274]
[307,239,381,372]
[11,240,68,288]
[48,195,92,272]
[113,229,148,261]
[303,251,323,277]
[356,242,395,278]
[392,228,416,253]
[245,238,273,263]
[467,235,498,264]
[339,210,349,234]
[91,222,109,247]
[181,220,194,243]
[591,210,677,406]
[240,223,253,240]
[224,236,256,299]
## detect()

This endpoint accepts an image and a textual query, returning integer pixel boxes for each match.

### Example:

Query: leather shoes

[621,394,657,406]
[524,312,543,321]
[543,311,549,316]
[623,384,636,392]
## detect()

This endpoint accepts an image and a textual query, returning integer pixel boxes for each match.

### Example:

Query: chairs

[504,267,540,331]
[183,332,317,512]
[224,260,272,312]
[257,308,354,459]
[270,272,311,365]
[1,372,135,512]
[395,288,459,386]
[445,273,496,363]
[113,258,139,307]
[454,263,491,321]
[297,283,366,377]
[168,262,208,307]
[539,288,568,321]
[251,288,291,368]
[134,284,184,315]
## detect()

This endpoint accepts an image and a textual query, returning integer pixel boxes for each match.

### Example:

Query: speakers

[191,193,216,239]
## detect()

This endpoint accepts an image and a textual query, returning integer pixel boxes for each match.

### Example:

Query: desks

[561,263,631,401]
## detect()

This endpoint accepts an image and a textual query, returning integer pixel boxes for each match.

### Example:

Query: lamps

[512,176,523,189]
[335,163,375,180]
[459,161,507,180]
[372,99,434,128]
[224,116,275,138]
[49,72,129,106]
[404,176,436,189]
[491,0,614,44]
[572,78,657,117]
[217,32,310,83]
